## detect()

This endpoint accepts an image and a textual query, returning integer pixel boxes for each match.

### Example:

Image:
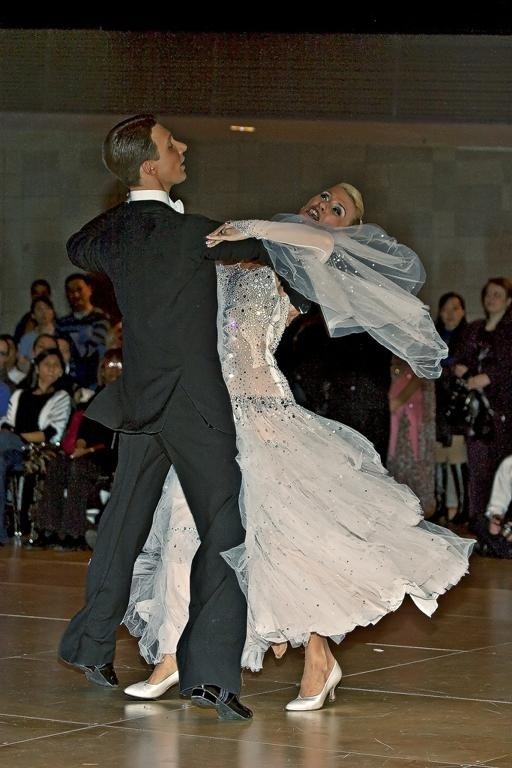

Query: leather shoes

[80,662,119,687]
[191,685,253,720]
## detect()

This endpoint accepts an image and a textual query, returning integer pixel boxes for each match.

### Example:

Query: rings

[219,228,226,236]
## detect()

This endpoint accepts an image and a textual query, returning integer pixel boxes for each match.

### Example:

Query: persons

[276,278,509,559]
[55,113,317,722]
[0,278,121,550]
[120,179,478,712]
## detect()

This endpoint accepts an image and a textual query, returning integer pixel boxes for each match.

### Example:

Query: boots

[425,462,449,524]
[450,463,470,524]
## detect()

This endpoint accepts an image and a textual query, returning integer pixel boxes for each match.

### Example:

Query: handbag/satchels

[471,522,507,559]
[446,378,494,437]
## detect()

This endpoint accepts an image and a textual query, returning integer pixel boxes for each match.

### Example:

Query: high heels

[285,660,342,711]
[124,671,179,698]
